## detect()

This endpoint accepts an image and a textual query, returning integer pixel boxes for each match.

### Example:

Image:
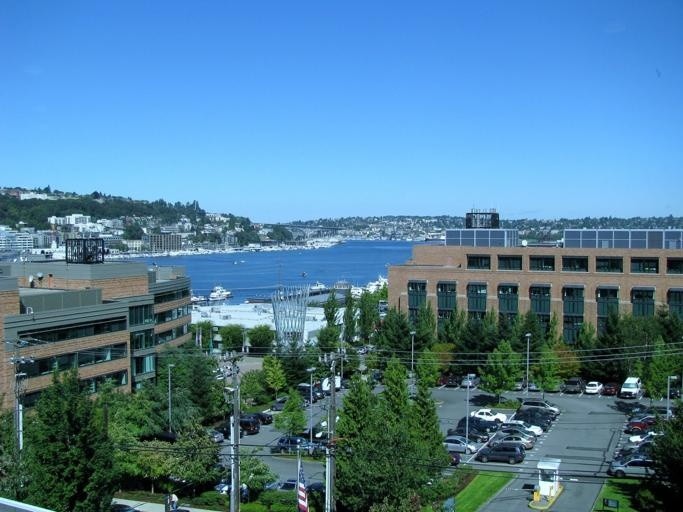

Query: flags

[298,461,308,512]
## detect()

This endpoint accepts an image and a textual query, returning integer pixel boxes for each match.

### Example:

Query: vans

[620,376,642,398]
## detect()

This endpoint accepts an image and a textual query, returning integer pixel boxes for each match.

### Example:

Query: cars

[213,478,299,503]
[511,377,619,396]
[271,428,330,457]
[435,375,481,387]
[608,408,673,480]
[204,411,273,442]
[442,398,560,465]
[270,383,327,412]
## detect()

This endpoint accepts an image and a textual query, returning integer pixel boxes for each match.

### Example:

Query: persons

[170,493,178,511]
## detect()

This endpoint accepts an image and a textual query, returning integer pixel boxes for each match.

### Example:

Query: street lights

[667,376,677,421]
[467,374,476,455]
[168,364,175,432]
[305,367,317,443]
[525,333,531,395]
[410,331,416,371]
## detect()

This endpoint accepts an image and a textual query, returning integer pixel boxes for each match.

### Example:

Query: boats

[191,285,233,303]
[310,276,388,301]
